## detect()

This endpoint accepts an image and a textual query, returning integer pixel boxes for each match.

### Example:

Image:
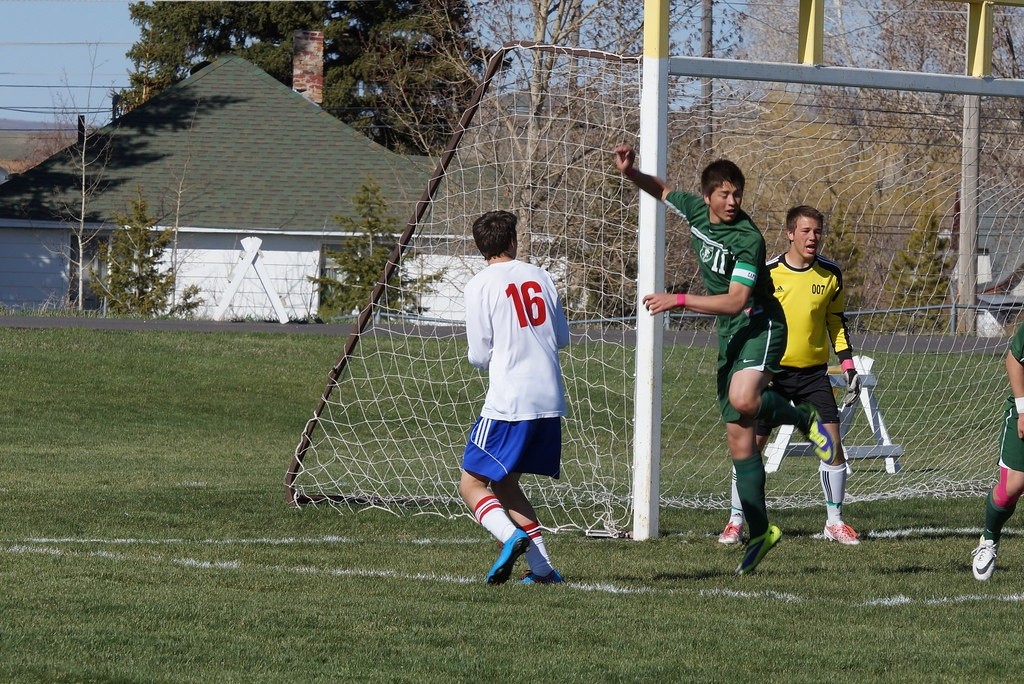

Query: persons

[614,144,835,576]
[716,203,861,545]
[459,210,569,583]
[969,320,1024,581]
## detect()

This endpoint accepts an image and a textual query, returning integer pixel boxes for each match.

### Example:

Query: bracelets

[1014,397,1024,414]
[676,294,686,307]
[840,359,855,374]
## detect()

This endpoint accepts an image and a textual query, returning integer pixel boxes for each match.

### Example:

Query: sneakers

[970,535,999,581]
[735,522,782,575]
[485,529,530,585]
[824,520,860,546]
[718,520,744,545]
[514,569,563,587]
